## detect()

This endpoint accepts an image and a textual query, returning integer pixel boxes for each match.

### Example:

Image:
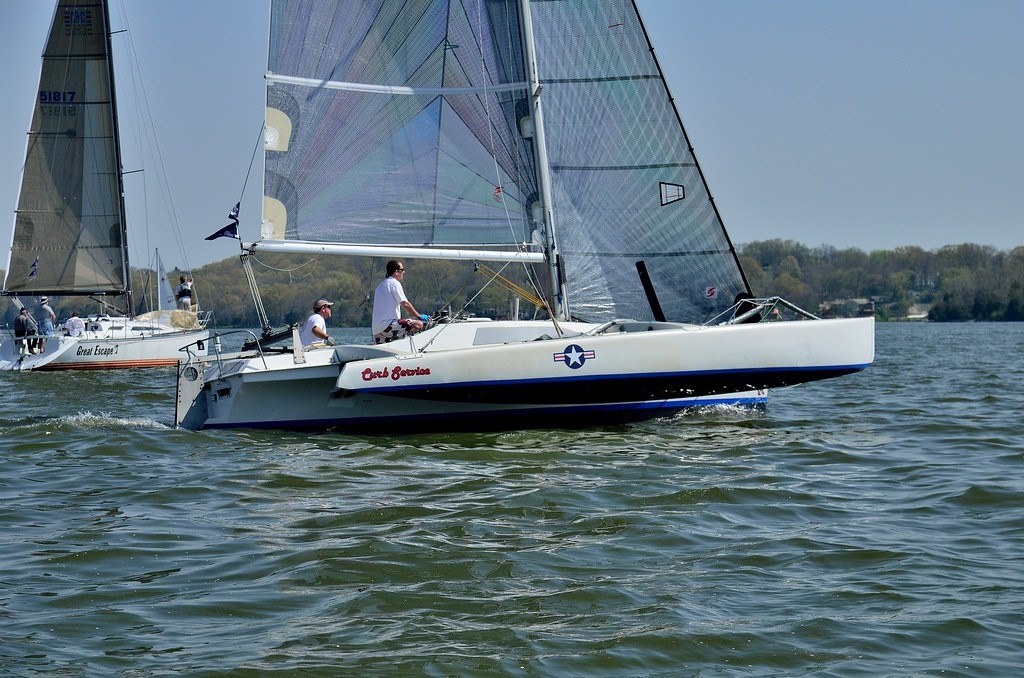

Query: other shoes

[29,349,37,355]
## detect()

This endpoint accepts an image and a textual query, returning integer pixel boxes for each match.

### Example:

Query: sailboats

[173,0,875,432]
[0,0,214,373]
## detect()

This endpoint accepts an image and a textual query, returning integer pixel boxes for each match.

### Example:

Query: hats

[313,299,334,310]
[180,275,187,281]
[39,296,48,304]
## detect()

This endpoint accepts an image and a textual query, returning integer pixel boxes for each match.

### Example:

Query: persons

[372,259,432,345]
[300,298,336,347]
[175,274,194,312]
[64,312,85,337]
[36,296,56,354]
[14,307,38,355]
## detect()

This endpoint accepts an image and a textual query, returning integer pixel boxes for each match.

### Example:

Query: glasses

[399,268,404,271]
[327,306,330,309]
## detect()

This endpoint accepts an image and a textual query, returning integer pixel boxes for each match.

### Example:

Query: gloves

[327,337,335,345]
